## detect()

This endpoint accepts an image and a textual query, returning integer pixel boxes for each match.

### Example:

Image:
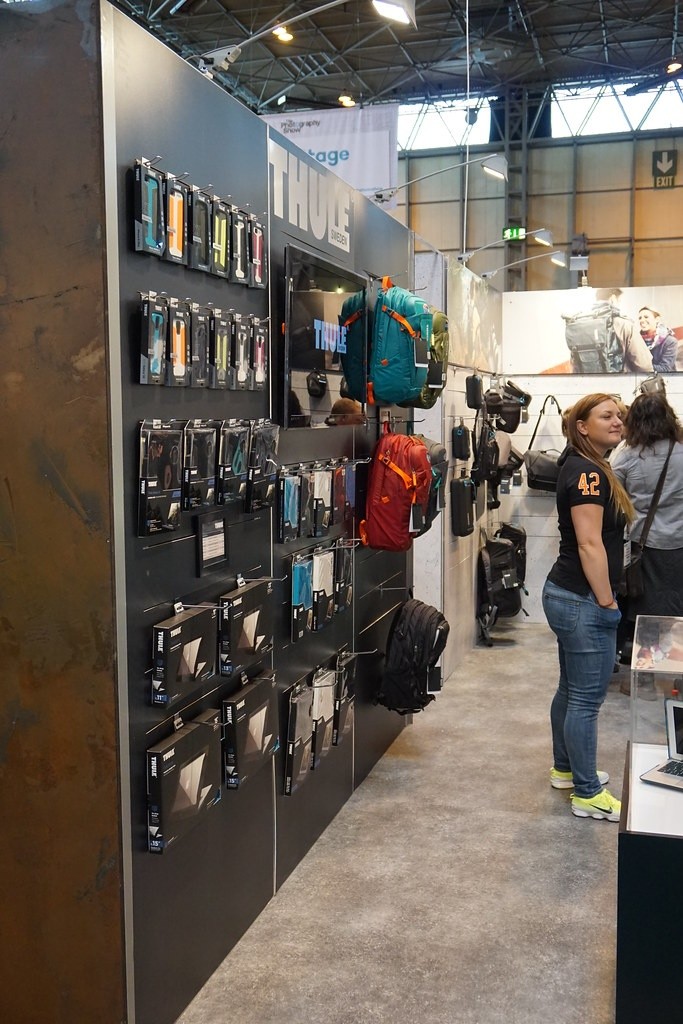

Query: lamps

[337,88,353,102]
[667,41,682,70]
[568,253,590,287]
[456,227,553,262]
[198,0,418,79]
[272,19,287,36]
[373,152,509,203]
[480,248,568,281]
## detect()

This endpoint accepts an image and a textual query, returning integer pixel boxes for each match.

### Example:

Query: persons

[635,307,680,373]
[574,288,654,371]
[540,391,638,822]
[609,394,683,701]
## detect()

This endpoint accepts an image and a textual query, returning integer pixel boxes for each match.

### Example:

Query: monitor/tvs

[275,242,370,430]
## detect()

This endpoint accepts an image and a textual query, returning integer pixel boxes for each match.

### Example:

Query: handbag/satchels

[617,554,643,597]
[306,371,326,398]
[451,467,475,537]
[523,449,564,492]
[470,380,532,509]
[339,376,356,402]
[452,417,470,461]
[465,374,484,410]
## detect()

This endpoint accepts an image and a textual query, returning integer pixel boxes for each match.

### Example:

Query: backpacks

[395,302,449,408]
[402,420,450,538]
[562,304,626,374]
[477,527,521,646]
[374,588,450,716]
[336,289,371,404]
[493,521,527,589]
[367,276,434,405]
[360,420,433,552]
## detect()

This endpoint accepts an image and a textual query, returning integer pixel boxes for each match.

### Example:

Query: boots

[619,673,658,700]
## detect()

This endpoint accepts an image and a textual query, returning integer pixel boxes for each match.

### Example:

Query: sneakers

[569,787,622,822]
[549,767,609,789]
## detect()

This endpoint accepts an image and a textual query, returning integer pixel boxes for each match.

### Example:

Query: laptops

[640,698,683,792]
